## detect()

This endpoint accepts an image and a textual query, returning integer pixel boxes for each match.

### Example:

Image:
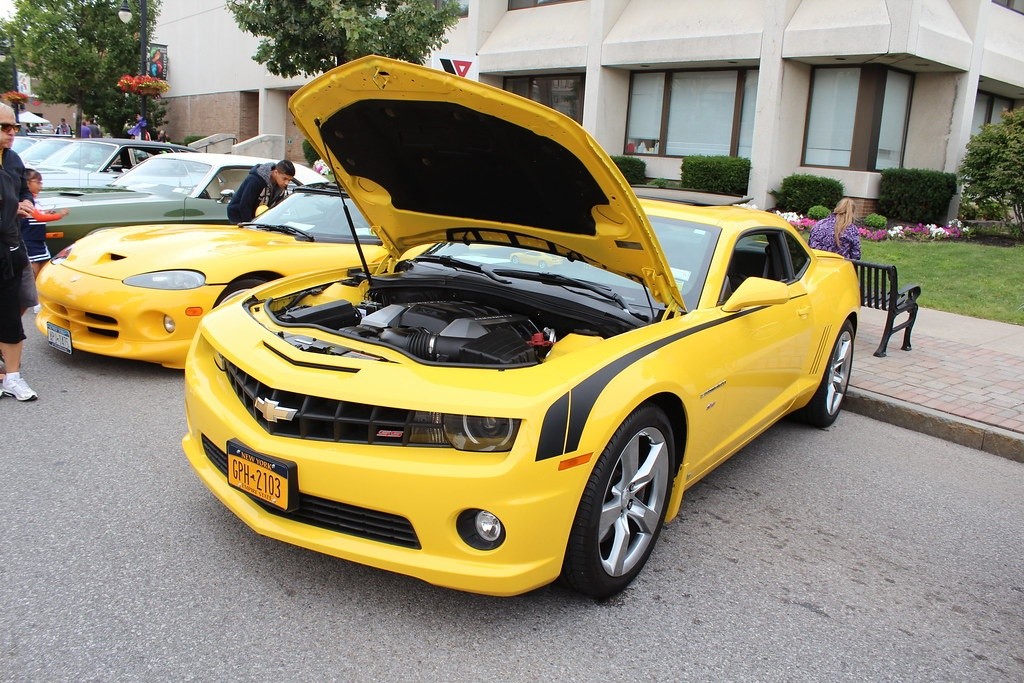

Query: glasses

[0,123,22,133]
[30,181,43,185]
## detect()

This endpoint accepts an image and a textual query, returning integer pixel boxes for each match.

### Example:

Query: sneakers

[0,377,38,401]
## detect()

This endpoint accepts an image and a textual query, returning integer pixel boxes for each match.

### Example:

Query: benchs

[845,258,921,358]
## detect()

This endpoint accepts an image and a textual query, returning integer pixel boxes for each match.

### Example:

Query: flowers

[115,70,171,101]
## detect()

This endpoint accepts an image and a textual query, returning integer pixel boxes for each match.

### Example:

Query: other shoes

[33,304,41,313]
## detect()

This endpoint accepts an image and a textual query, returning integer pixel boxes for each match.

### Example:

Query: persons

[150,49,163,77]
[226,159,295,225]
[0,102,100,402]
[807,197,861,260]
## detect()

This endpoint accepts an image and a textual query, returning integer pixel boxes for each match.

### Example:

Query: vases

[139,87,159,96]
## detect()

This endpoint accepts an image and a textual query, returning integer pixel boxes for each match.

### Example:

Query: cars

[33,153,331,260]
[35,188,437,369]
[10,136,227,198]
[181,55,861,597]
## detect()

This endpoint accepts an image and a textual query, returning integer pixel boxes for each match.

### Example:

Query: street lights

[117,0,171,141]
[0,49,28,121]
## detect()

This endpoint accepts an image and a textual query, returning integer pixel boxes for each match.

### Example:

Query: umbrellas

[19,111,50,127]
